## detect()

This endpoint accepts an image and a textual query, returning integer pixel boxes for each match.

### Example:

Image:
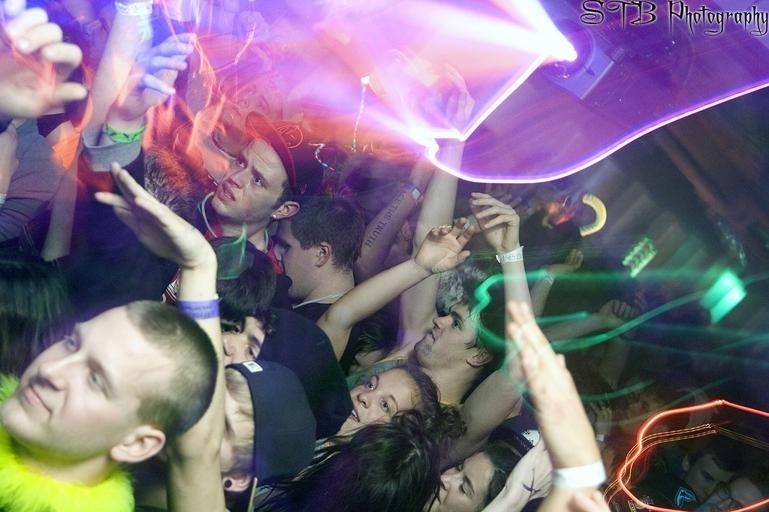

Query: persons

[1,1,769,511]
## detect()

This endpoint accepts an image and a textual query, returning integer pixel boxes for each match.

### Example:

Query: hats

[227,308,354,512]
[246,112,328,201]
